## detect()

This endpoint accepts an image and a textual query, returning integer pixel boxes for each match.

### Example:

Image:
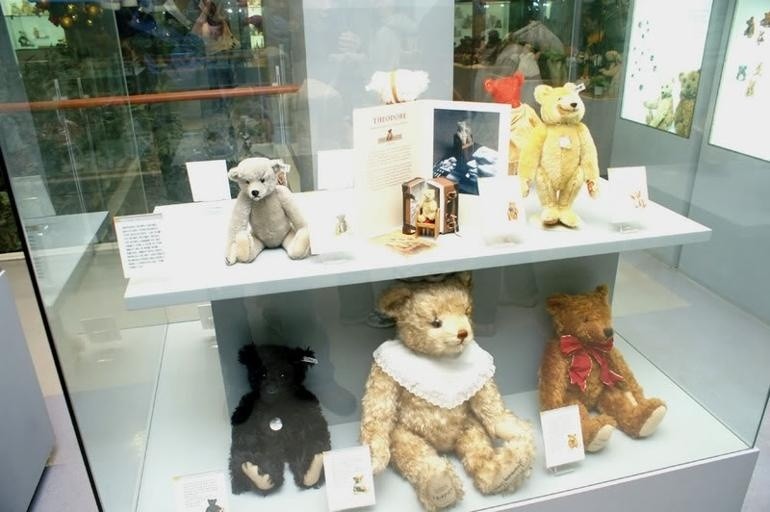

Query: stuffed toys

[484,71,542,177]
[360,270,535,512]
[416,188,438,223]
[223,156,311,266]
[228,341,332,497]
[537,282,668,454]
[518,80,601,230]
[642,77,676,130]
[673,69,701,137]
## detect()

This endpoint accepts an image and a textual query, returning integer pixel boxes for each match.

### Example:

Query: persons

[386,128,393,141]
[191,0,247,153]
[460,6,622,97]
[454,121,473,173]
